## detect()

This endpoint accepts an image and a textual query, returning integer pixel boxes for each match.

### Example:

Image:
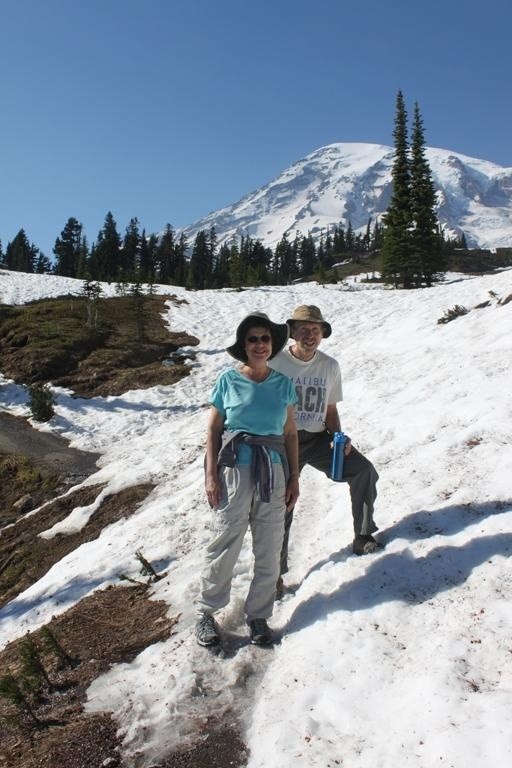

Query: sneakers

[276,580,296,600]
[193,615,220,647]
[354,536,385,556]
[245,619,273,645]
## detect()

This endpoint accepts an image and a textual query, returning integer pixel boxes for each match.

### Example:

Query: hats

[286,305,332,339]
[225,312,290,363]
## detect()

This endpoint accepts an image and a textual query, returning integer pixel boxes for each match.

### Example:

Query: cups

[331,431,346,482]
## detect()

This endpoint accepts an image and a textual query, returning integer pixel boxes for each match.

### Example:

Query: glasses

[244,334,272,343]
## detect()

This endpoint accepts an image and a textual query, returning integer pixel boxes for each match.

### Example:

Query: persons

[272,303,387,603]
[192,307,301,650]
